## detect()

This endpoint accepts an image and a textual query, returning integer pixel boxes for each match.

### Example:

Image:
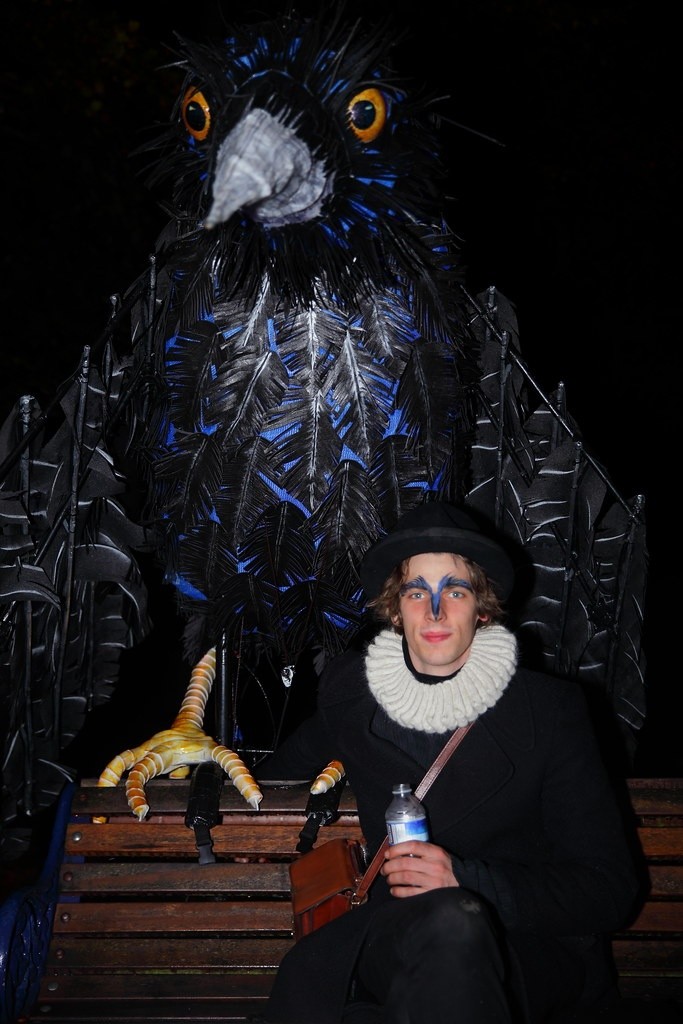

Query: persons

[262,496,652,1022]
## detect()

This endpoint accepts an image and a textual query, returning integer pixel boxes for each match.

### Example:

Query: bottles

[386,784,429,887]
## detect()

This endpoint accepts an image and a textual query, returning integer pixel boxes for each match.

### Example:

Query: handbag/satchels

[288,839,369,944]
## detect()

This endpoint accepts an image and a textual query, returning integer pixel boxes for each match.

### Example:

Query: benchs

[25,773,681,1024]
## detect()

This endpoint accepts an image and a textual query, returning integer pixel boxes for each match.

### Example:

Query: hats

[359,499,513,603]
